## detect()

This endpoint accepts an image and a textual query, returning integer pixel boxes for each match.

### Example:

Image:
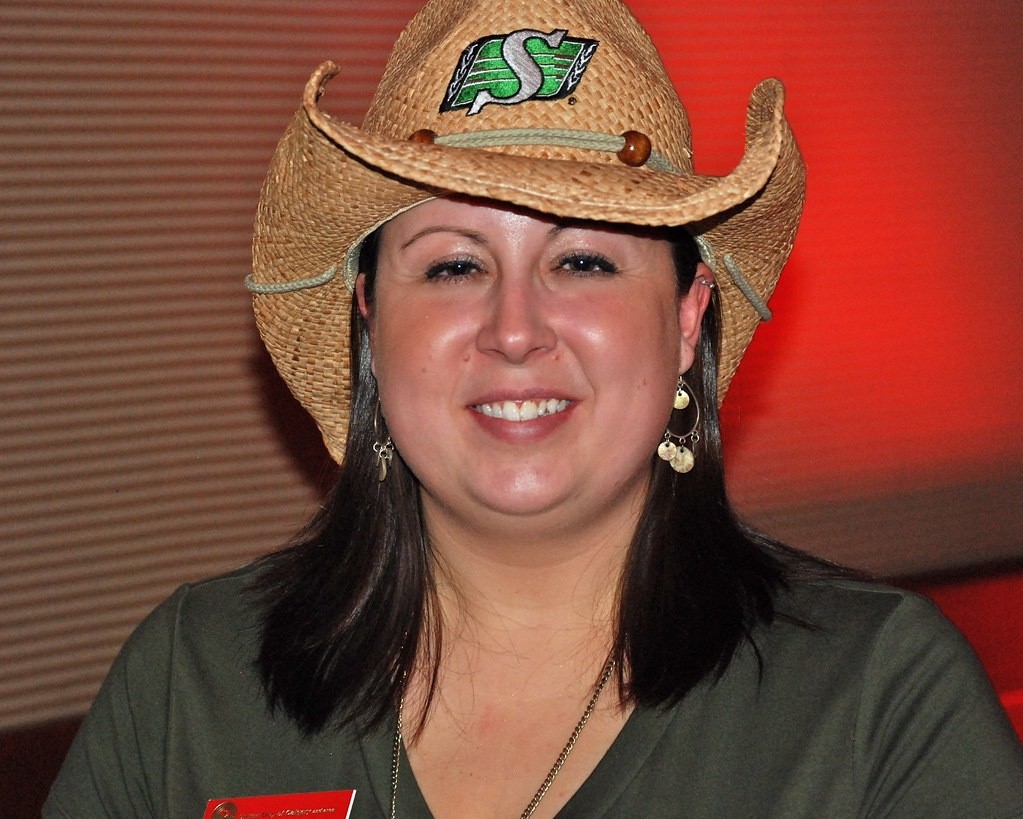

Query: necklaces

[391,637,617,818]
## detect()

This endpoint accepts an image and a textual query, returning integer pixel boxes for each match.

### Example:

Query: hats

[246,1,805,468]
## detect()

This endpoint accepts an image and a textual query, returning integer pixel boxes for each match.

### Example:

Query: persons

[42,0,1023,818]
